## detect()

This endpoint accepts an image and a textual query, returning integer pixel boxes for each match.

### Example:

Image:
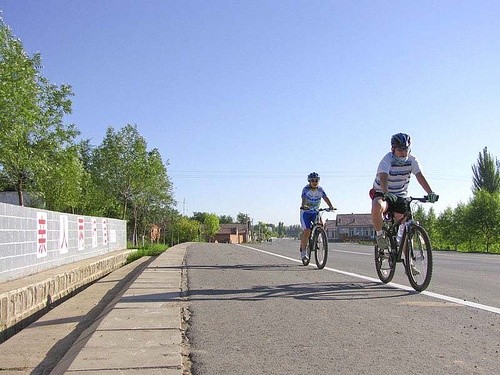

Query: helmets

[307,172,320,180]
[391,133,411,146]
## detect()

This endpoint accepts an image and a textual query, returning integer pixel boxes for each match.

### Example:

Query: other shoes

[301,254,309,260]
[404,265,421,275]
[375,234,388,249]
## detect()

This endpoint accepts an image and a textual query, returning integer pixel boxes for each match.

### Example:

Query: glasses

[395,147,409,151]
[311,180,318,183]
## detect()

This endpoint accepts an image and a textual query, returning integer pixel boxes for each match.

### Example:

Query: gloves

[384,192,397,203]
[428,192,437,203]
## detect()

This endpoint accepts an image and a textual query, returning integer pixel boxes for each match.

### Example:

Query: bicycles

[373,194,439,292]
[300,206,337,269]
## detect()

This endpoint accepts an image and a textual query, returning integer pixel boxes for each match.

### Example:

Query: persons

[256,236,272,243]
[371,133,437,275]
[300,172,334,259]
